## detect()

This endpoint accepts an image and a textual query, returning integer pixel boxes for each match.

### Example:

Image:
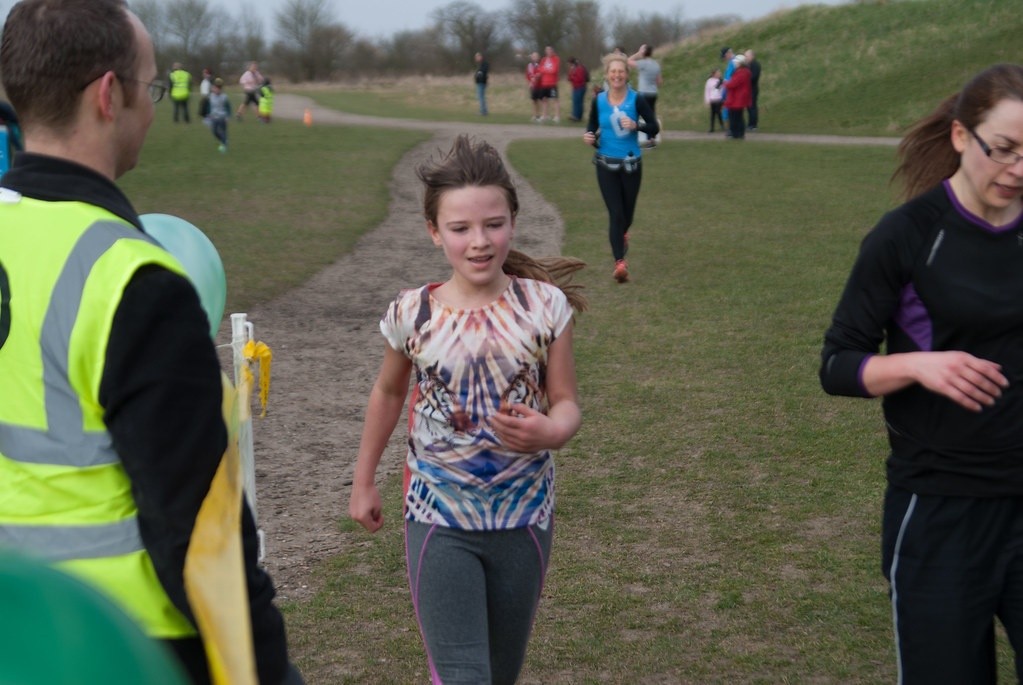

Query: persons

[582,53,659,283]
[198,66,214,117]
[205,78,231,152]
[627,44,662,149]
[348,133,590,685]
[255,77,273,123]
[0,0,304,685]
[704,68,726,133]
[168,62,193,121]
[535,45,561,123]
[819,64,1023,685]
[526,51,541,121]
[474,50,489,117]
[723,54,752,139]
[566,55,586,121]
[719,46,736,135]
[744,49,760,131]
[234,60,263,119]
[613,45,624,53]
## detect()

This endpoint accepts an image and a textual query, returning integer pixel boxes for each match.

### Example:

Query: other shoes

[642,140,657,149]
[218,144,227,152]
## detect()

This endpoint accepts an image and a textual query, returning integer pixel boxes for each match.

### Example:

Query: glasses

[963,122,1022,165]
[80,69,167,103]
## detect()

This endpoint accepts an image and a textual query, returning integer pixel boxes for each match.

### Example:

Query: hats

[721,47,729,59]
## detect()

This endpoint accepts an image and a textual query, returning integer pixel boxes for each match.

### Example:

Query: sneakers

[624,232,631,255]
[612,262,629,284]
